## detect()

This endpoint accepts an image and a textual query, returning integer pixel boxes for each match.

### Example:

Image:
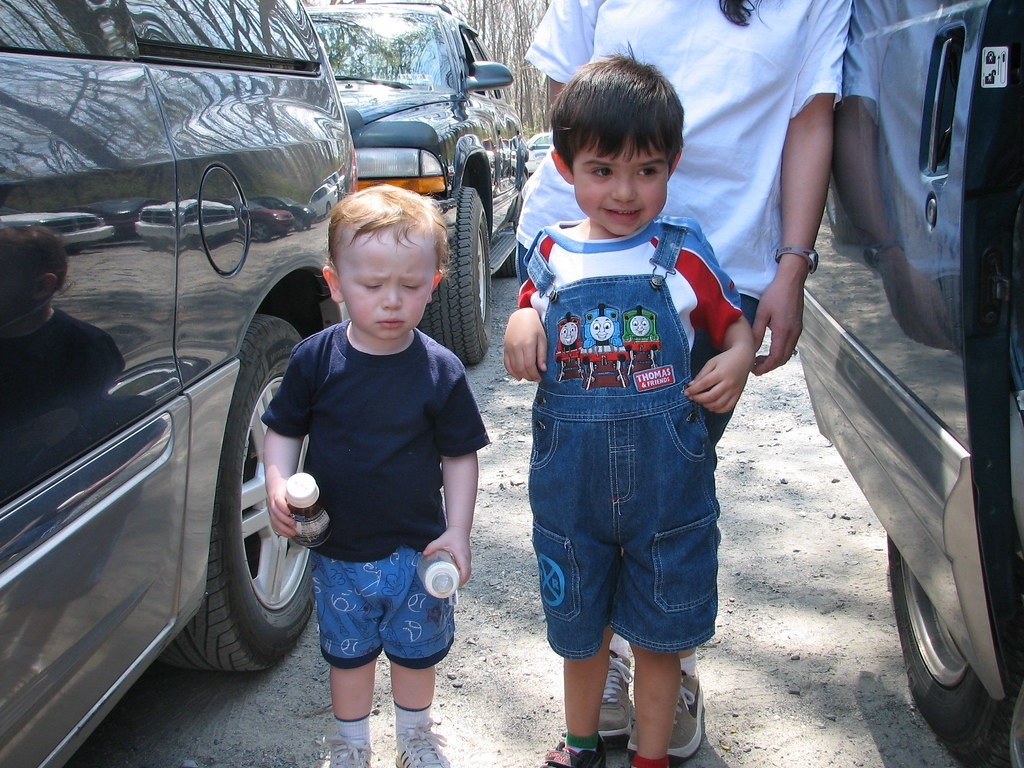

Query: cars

[306,164,348,221]
[525,131,553,177]
[0,204,114,256]
[62,196,316,251]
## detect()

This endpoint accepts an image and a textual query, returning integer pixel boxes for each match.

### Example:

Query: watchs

[775,246,818,275]
[863,239,897,268]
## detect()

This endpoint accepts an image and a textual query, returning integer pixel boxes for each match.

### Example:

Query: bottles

[286,472,332,548]
[417,549,460,599]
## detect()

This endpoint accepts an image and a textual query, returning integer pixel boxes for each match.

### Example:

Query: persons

[831,1,960,354]
[0,224,154,693]
[512,0,851,765]
[501,42,754,768]
[258,184,489,768]
[491,130,525,196]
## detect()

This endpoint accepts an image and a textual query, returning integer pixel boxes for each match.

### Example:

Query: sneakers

[325,734,372,768]
[627,666,703,761]
[539,733,606,768]
[396,718,451,768]
[598,649,632,736]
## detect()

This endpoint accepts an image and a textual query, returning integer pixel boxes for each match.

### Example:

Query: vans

[799,0,1022,768]
[0,1,358,768]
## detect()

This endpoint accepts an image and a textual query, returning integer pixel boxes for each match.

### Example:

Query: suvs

[306,2,527,364]
